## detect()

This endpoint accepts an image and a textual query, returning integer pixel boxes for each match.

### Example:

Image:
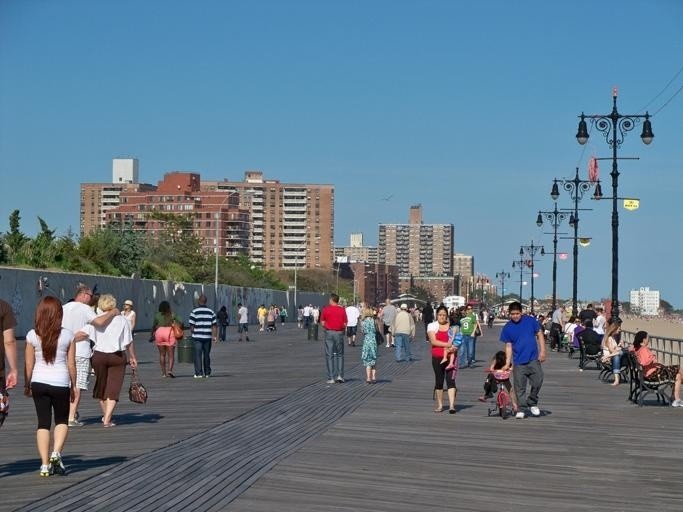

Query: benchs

[556,328,675,407]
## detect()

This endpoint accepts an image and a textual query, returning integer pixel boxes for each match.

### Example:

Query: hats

[122,300,133,306]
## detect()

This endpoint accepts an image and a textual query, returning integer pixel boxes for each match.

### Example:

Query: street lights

[512,257,530,301]
[336,253,356,297]
[214,191,237,317]
[535,202,574,313]
[577,86,656,343]
[519,240,546,311]
[353,264,371,306]
[294,236,320,321]
[550,166,604,317]
[495,268,510,297]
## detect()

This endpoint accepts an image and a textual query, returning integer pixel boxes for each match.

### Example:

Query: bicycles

[487,317,494,328]
[482,367,516,419]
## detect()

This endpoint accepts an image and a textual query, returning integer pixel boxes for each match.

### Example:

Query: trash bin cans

[178,337,195,363]
[308,324,318,340]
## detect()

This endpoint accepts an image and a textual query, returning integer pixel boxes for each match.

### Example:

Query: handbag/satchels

[129,382,148,404]
[375,331,384,345]
[172,324,184,338]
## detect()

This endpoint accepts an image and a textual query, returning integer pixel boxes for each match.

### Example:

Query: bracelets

[24,383,31,388]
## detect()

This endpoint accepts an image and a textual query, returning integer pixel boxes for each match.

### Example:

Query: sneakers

[39,463,55,477]
[326,379,335,384]
[516,412,525,419]
[530,406,540,415]
[672,398,683,407]
[50,452,66,475]
[168,371,176,377]
[194,375,202,379]
[337,377,345,384]
[68,420,84,427]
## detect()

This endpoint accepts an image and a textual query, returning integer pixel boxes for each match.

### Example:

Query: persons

[391,303,415,362]
[152,301,183,379]
[479,307,494,328]
[320,294,348,385]
[62,283,138,428]
[480,351,517,411]
[0,275,18,427]
[457,305,482,369]
[600,322,683,407]
[295,295,466,348]
[216,305,229,342]
[427,302,462,413]
[24,296,76,477]
[500,302,546,418]
[238,304,249,341]
[257,304,288,332]
[187,295,218,378]
[361,307,380,384]
[533,304,606,353]
